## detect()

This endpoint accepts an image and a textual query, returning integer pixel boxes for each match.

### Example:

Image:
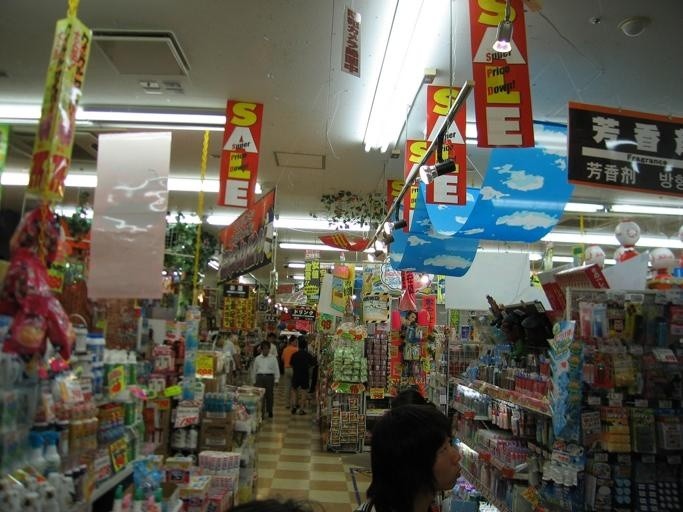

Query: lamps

[418,159,455,185]
[493,21,515,51]
[618,15,651,38]
[382,237,393,247]
[160,259,219,279]
[367,250,384,263]
[384,220,407,235]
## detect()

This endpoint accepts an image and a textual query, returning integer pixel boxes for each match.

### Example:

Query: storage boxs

[199,413,236,451]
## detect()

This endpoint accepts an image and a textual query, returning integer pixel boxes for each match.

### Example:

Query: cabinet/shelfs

[360,391,397,452]
[425,288,683,511]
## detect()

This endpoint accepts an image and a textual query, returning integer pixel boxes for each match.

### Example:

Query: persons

[222,496,322,511]
[350,403,463,511]
[210,330,316,421]
[400,310,417,328]
[391,387,436,408]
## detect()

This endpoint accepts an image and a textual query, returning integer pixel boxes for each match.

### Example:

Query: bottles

[112,484,164,512]
[203,391,232,413]
[102,348,138,391]
[3,464,88,511]
[97,416,125,448]
